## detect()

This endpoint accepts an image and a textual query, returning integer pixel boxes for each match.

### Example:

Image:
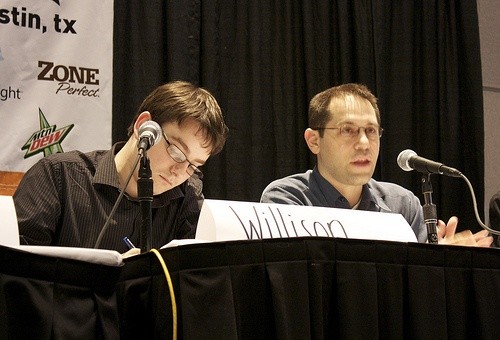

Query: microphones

[138,121,163,157]
[396,149,463,179]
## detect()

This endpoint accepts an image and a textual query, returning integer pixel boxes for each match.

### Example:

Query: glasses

[311,124,383,139]
[161,125,203,180]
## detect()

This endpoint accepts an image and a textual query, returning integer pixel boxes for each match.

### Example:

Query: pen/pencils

[123,237,136,250]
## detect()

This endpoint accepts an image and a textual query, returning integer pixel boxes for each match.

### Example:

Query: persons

[260,83,494,248]
[12,81,228,259]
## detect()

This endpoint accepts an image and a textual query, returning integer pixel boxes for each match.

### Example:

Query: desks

[0,230,499,340]
